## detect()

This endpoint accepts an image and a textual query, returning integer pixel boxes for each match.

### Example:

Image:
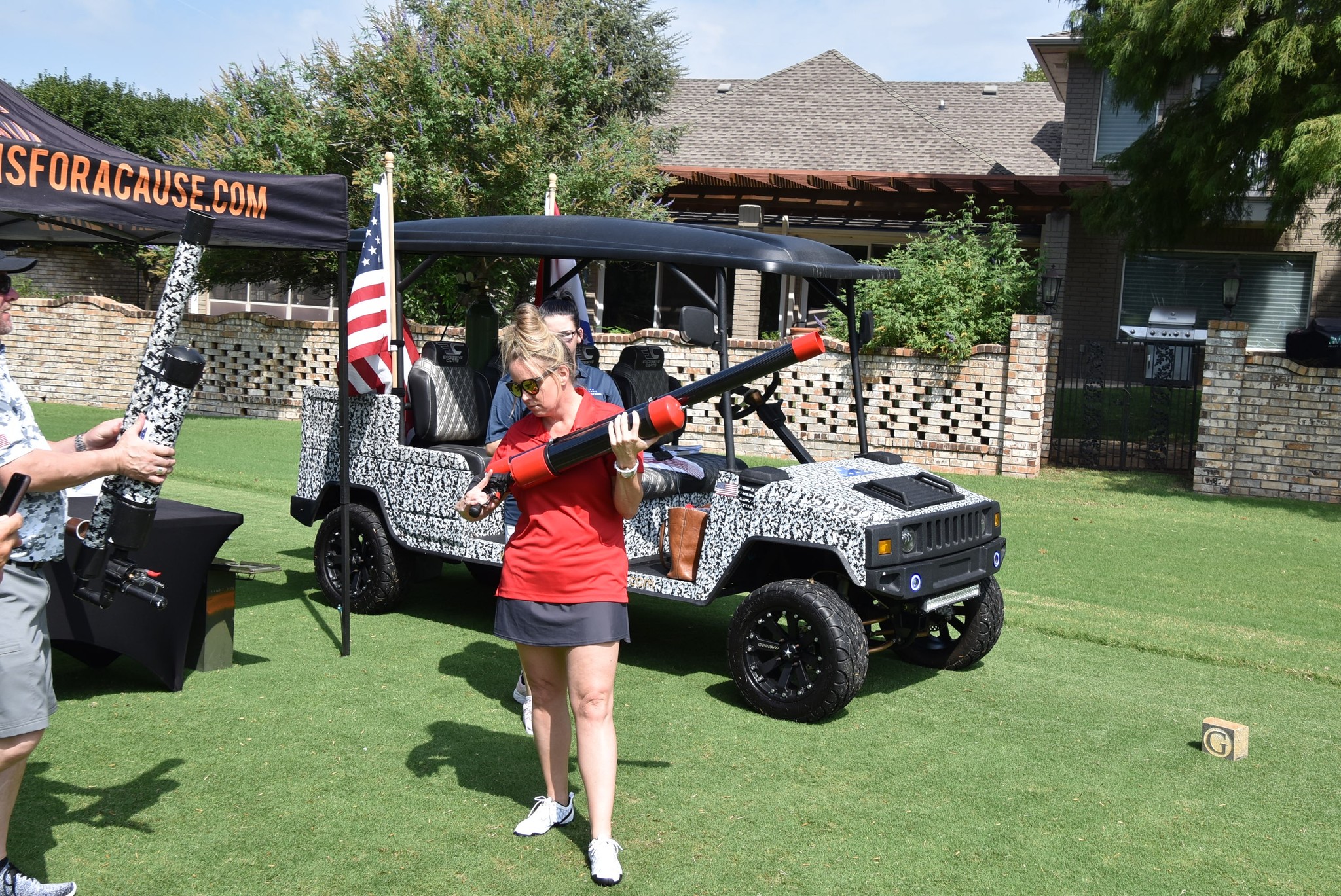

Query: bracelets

[75,432,89,452]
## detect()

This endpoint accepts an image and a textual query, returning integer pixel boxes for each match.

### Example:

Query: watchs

[614,459,640,480]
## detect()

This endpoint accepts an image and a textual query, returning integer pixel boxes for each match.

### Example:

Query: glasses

[0,275,13,296]
[557,330,579,343]
[505,364,560,397]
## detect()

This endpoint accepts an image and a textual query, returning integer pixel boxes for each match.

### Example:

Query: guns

[465,331,827,518]
[67,208,217,609]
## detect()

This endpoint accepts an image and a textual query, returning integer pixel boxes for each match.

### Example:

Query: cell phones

[0,473,31,517]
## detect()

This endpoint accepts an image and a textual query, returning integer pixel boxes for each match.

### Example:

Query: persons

[0,511,25,579]
[457,303,665,887]
[484,290,623,739]
[0,252,176,896]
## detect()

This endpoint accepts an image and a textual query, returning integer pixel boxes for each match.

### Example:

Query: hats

[0,249,40,274]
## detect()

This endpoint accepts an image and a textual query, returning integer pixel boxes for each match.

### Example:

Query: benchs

[577,345,748,499]
[409,341,509,476]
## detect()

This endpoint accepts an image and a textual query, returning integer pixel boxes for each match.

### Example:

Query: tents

[0,78,354,659]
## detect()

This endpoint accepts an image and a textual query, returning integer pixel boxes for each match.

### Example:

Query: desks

[43,496,243,692]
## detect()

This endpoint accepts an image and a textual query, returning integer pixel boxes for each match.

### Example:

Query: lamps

[1223,265,1245,317]
[1041,265,1063,316]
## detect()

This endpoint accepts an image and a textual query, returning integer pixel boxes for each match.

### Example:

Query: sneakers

[513,792,575,836]
[513,670,526,704]
[0,860,77,896]
[587,836,623,886]
[522,695,533,735]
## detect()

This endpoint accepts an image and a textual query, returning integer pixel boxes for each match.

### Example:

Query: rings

[157,466,163,475]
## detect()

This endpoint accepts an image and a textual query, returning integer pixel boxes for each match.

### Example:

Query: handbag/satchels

[657,501,713,584]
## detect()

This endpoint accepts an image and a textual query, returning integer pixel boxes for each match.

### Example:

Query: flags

[334,181,422,446]
[532,188,597,365]
